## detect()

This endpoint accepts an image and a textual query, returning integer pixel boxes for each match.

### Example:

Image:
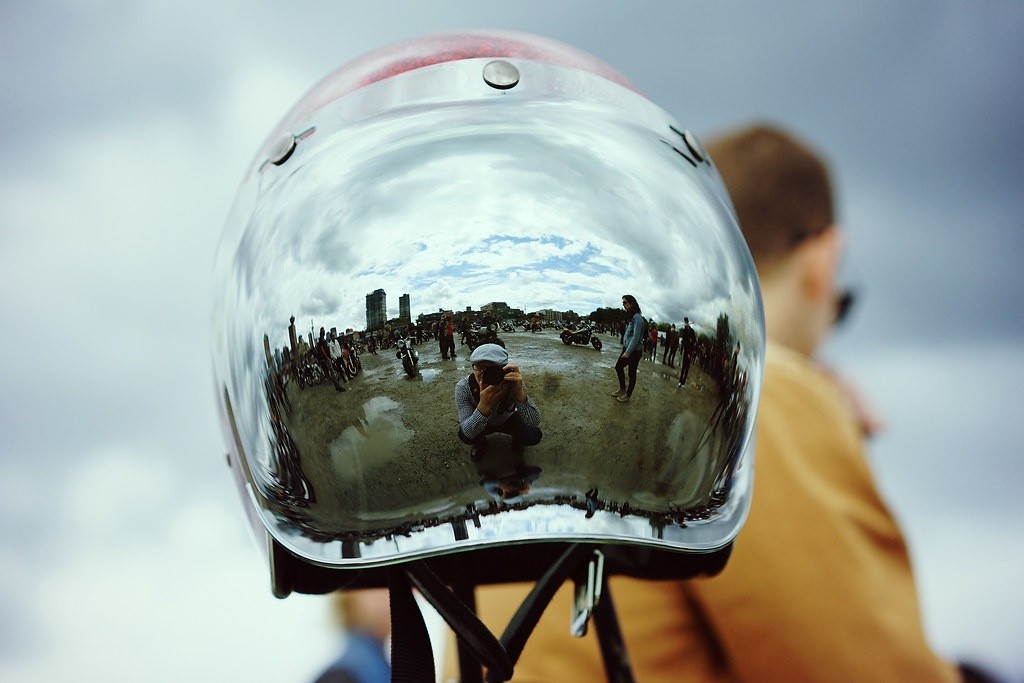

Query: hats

[469,343,509,365]
[698,125,834,261]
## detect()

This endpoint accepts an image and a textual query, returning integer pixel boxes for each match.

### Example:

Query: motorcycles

[559,320,602,351]
[352,328,439,355]
[464,322,500,351]
[501,321,606,333]
[295,346,361,390]
[394,332,419,378]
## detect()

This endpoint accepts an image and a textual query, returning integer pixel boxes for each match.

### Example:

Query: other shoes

[335,386,346,392]
[470,436,487,462]
[511,435,525,455]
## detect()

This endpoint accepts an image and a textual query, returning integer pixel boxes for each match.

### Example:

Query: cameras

[483,363,512,385]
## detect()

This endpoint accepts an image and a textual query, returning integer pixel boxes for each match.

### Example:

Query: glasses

[622,301,631,305]
[472,363,490,372]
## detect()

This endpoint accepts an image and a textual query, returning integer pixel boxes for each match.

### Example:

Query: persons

[612,294,648,402]
[261,318,425,418]
[308,586,399,683]
[464,494,579,518]
[603,489,725,529]
[610,316,748,437]
[431,312,504,361]
[447,129,962,683]
[455,343,542,461]
[585,486,601,520]
[317,516,441,539]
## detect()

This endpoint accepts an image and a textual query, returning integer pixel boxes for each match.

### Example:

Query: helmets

[209,30,763,579]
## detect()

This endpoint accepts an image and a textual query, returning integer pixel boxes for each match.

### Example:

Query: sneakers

[612,387,627,396]
[617,393,629,402]
